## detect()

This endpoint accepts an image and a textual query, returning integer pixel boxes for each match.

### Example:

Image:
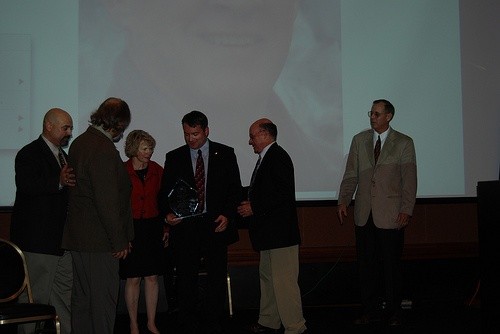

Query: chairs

[0,239,61,334]
[468,180,500,306]
[171,239,234,319]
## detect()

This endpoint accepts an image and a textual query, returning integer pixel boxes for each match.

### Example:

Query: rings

[66,180,69,183]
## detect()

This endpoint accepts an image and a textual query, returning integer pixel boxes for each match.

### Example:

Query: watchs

[164,213,168,224]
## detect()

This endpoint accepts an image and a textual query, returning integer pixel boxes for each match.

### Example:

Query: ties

[58,147,66,169]
[251,154,261,182]
[194,149,205,214]
[374,136,381,165]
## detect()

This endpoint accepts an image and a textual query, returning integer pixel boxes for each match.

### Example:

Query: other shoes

[244,323,272,334]
[390,319,401,328]
[355,314,368,325]
[146,325,161,334]
[301,330,310,334]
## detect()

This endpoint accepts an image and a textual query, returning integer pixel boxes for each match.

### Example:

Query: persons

[338,99,417,326]
[238,118,307,334]
[60,97,134,334]
[10,108,75,333]
[120,130,169,334]
[156,110,242,334]
